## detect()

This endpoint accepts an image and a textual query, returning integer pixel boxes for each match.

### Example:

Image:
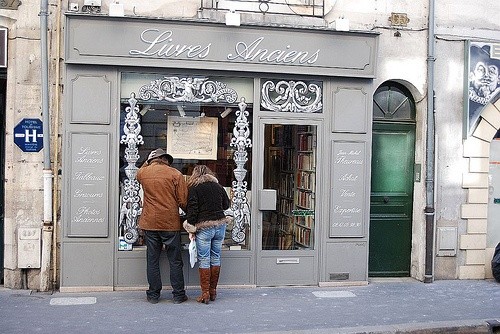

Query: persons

[137,149,188,305]
[182,164,230,304]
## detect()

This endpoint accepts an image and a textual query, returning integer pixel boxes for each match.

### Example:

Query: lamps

[176,105,185,116]
[139,104,153,115]
[221,108,233,117]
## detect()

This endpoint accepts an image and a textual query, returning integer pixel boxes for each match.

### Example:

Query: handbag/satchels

[189,238,198,269]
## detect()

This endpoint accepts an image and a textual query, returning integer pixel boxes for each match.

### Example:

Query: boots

[196,266,221,304]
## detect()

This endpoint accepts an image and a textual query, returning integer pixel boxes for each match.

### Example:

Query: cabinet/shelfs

[270,125,315,249]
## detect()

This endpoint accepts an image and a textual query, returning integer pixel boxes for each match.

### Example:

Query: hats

[147,148,174,163]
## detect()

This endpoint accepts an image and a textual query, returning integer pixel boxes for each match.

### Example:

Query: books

[263,125,317,250]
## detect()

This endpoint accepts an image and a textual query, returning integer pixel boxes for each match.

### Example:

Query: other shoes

[174,295,188,304]
